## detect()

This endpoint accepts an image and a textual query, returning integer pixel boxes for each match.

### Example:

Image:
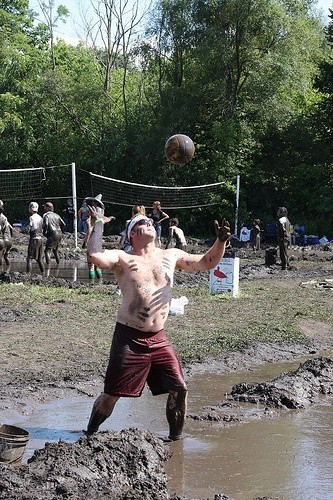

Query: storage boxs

[209,256,240,299]
[268,222,328,246]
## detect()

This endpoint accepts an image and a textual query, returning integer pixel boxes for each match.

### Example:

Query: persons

[23,202,45,274]
[276,207,291,270]
[87,205,232,440]
[293,223,305,247]
[165,218,187,252]
[148,201,169,244]
[79,194,147,285]
[41,203,66,264]
[253,219,264,251]
[0,199,13,266]
[61,199,75,233]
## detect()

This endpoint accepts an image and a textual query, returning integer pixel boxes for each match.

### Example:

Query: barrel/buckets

[265,249,278,266]
[0,424,29,462]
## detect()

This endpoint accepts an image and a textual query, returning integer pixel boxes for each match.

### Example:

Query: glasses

[136,218,153,225]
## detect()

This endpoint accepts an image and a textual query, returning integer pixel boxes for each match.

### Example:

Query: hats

[83,192,106,209]
[0,200,3,208]
[30,201,39,212]
[42,202,53,208]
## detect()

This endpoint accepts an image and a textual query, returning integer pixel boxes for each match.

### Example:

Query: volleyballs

[164,134,196,166]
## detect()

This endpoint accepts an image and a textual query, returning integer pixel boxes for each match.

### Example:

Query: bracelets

[94,218,103,223]
